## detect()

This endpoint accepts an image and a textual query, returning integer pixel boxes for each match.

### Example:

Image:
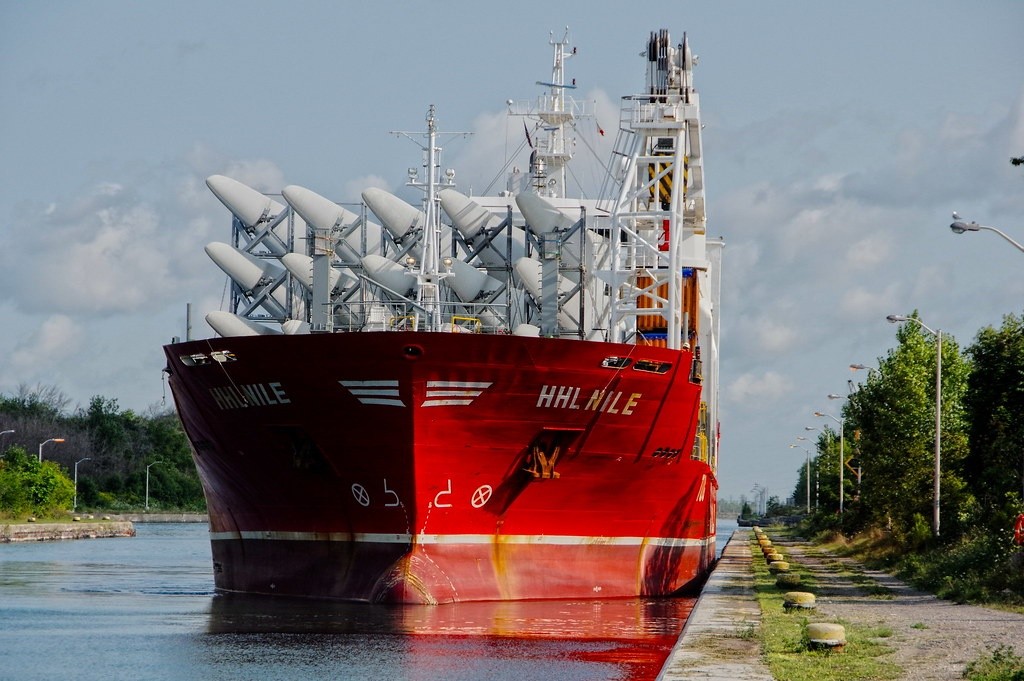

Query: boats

[162,24,726,606]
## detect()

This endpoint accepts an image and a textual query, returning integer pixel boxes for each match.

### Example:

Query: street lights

[790,426,831,516]
[750,482,770,518]
[814,412,844,515]
[74,458,92,509]
[37,438,64,467]
[886,313,942,536]
[145,461,163,510]
